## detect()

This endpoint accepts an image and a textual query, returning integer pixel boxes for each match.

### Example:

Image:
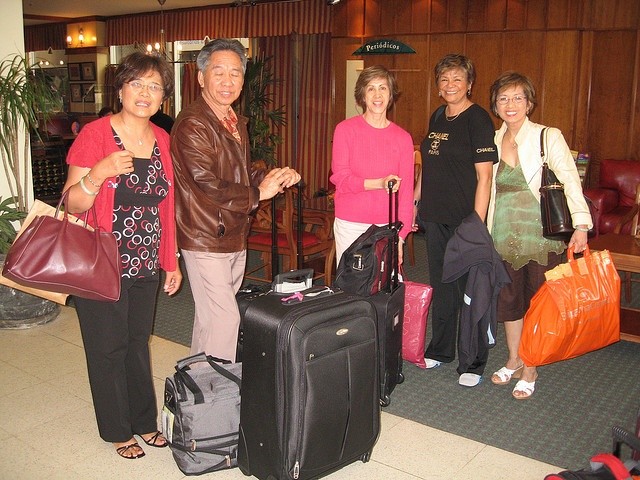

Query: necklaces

[504,132,518,149]
[444,101,467,122]
[119,112,151,147]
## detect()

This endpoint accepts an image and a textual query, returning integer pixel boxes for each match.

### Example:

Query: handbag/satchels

[1,185,121,303]
[163,351,242,476]
[517,244,621,367]
[544,454,640,480]
[334,221,405,297]
[0,199,94,306]
[539,127,600,241]
[397,264,433,371]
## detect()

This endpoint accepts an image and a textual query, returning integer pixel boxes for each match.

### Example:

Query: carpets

[65,231,640,471]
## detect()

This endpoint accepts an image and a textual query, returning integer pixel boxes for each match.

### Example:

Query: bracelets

[175,251,181,259]
[413,199,420,207]
[399,236,407,246]
[576,227,589,232]
[85,172,103,191]
[79,177,98,198]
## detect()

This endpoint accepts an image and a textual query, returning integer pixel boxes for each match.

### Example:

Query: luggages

[235,180,381,480]
[330,178,405,407]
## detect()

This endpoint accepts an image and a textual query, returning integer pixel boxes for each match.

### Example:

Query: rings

[580,245,587,250]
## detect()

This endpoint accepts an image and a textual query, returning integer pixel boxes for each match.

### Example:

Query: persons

[410,56,500,391]
[329,66,420,273]
[482,73,594,403]
[168,36,306,365]
[60,53,184,464]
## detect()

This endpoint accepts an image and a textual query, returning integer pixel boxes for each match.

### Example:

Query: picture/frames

[70,83,82,101]
[68,62,80,80]
[81,61,95,80]
[83,83,95,102]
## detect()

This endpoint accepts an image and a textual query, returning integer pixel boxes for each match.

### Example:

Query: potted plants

[1,43,65,329]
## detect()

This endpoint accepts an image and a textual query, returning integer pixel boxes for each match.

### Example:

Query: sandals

[116,443,146,459]
[137,431,169,448]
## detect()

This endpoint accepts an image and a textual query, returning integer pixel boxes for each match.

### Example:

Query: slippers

[511,380,536,399]
[491,365,524,385]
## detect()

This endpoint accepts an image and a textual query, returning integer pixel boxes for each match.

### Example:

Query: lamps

[66,21,106,49]
[145,0,166,58]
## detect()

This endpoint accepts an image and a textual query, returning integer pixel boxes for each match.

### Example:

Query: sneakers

[416,357,443,368]
[458,373,482,387]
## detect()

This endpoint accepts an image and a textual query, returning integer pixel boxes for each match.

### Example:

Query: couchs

[586,160,640,239]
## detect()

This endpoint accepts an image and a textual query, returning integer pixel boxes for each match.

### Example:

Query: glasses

[494,96,528,104]
[123,80,165,94]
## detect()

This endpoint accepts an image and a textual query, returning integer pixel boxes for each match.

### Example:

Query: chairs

[404,144,422,267]
[244,161,335,287]
